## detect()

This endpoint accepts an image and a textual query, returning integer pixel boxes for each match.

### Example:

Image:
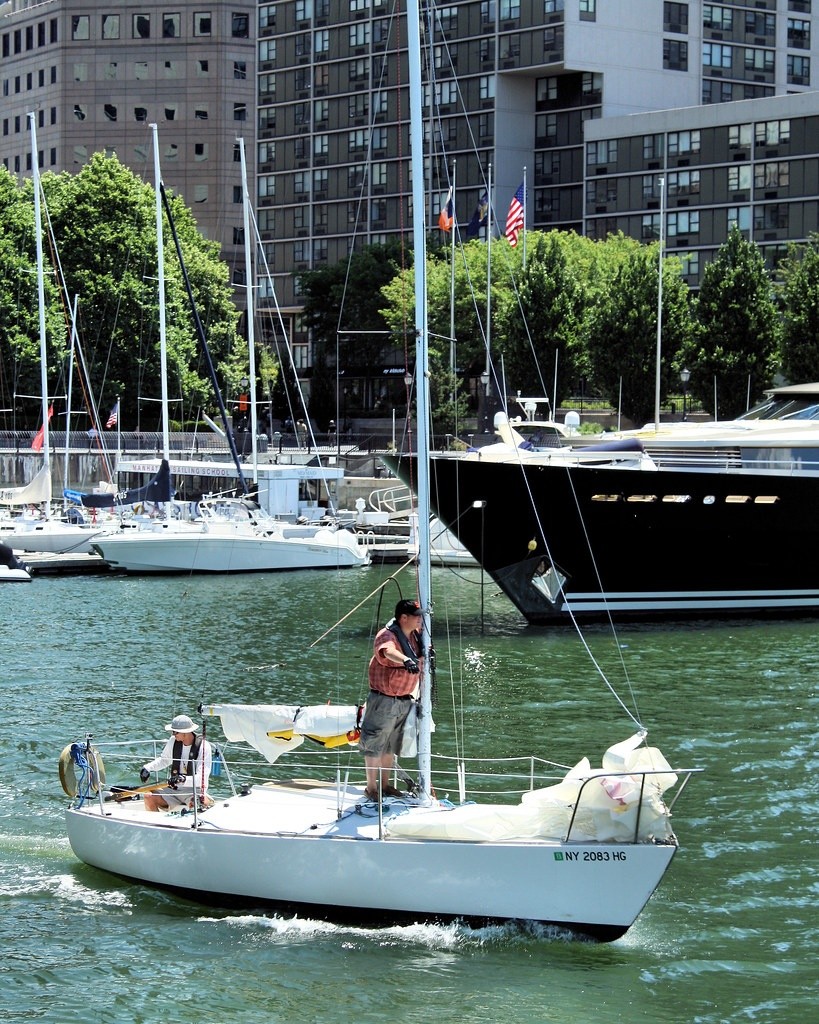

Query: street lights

[240,375,250,433]
[478,369,492,434]
[678,366,692,422]
[404,371,414,434]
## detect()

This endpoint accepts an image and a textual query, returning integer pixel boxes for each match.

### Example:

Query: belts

[370,689,415,700]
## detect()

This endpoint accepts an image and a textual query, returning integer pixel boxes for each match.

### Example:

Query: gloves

[403,658,420,674]
[140,768,150,783]
[168,775,186,785]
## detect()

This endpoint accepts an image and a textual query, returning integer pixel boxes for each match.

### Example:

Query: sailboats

[0,110,484,584]
[50,0,712,947]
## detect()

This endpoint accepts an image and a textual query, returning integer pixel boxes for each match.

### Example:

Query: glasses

[172,732,178,735]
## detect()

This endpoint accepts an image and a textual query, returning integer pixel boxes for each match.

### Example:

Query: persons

[140,715,212,811]
[357,599,427,803]
[327,420,336,448]
[297,418,307,449]
[285,416,294,433]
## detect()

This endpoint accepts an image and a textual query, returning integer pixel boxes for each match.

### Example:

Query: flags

[505,181,528,247]
[465,184,489,235]
[106,403,118,428]
[31,403,53,452]
[438,178,454,233]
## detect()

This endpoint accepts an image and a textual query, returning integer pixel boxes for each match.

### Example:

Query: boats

[378,113,818,633]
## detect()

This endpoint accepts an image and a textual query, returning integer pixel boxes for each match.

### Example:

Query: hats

[395,599,428,618]
[164,715,199,733]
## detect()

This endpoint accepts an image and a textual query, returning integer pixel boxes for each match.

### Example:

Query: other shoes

[377,785,405,797]
[364,787,390,813]
